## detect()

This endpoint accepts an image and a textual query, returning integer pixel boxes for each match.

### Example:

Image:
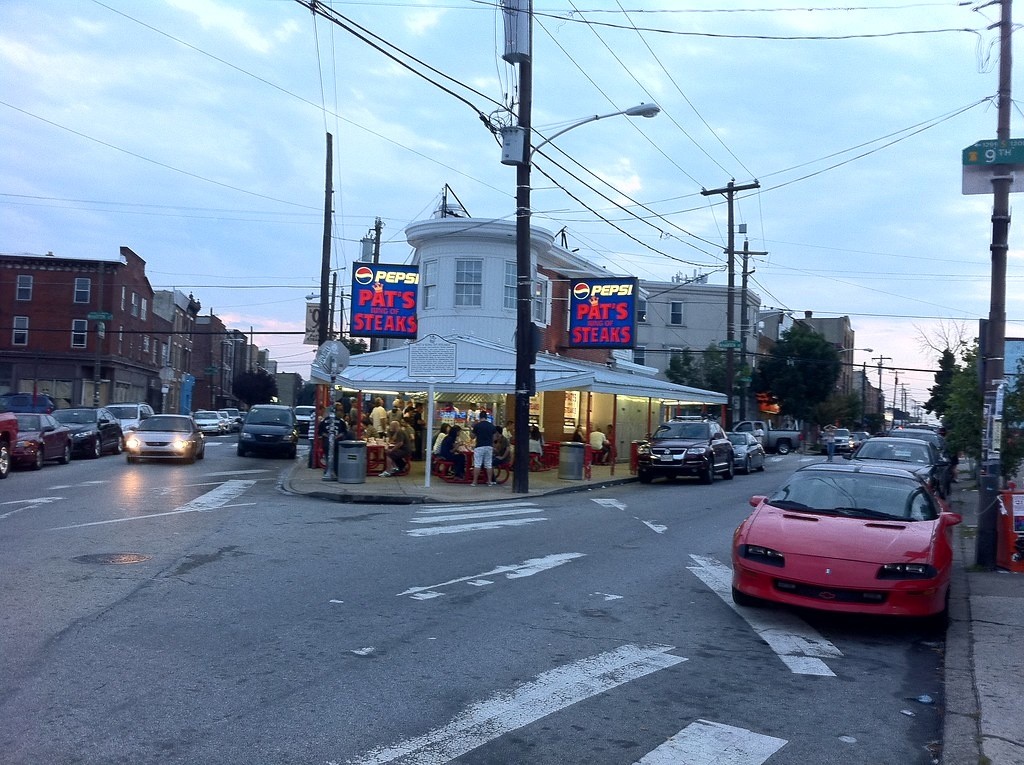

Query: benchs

[395,454,411,477]
[494,462,510,483]
[431,453,453,478]
[529,451,605,473]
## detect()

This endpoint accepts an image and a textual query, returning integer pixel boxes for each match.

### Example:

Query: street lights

[511,103,662,493]
[92,321,106,406]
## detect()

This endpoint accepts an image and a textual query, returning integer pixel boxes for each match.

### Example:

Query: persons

[529,425,545,468]
[306,392,426,478]
[572,424,587,443]
[824,424,838,462]
[782,423,792,430]
[491,420,514,484]
[605,423,615,445]
[440,424,466,480]
[446,401,459,412]
[467,401,491,421]
[755,423,763,430]
[433,422,451,459]
[470,410,497,487]
[590,427,611,465]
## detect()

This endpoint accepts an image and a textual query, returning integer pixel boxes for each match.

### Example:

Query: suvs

[636,414,735,485]
[103,401,155,451]
[0,392,55,415]
[819,428,852,455]
[234,404,303,459]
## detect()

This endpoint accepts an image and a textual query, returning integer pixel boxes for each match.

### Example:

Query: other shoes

[602,461,607,464]
[488,482,493,488]
[402,464,408,473]
[470,482,477,487]
[453,475,463,481]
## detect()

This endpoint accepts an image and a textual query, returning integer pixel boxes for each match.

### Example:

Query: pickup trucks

[725,420,804,455]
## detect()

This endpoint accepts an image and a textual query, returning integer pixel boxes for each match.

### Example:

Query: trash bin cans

[338,440,367,484]
[558,441,585,480]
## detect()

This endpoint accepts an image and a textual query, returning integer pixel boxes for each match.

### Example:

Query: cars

[292,406,317,428]
[0,407,19,479]
[50,406,125,460]
[842,436,949,503]
[127,414,206,465]
[9,412,74,470]
[886,426,948,469]
[849,422,947,454]
[723,432,767,475]
[192,408,248,436]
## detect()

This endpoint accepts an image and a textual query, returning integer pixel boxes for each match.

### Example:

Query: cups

[363,437,389,443]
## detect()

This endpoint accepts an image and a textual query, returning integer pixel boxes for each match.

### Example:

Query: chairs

[875,448,894,460]
[911,447,927,461]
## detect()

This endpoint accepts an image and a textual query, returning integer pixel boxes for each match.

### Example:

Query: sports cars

[731,463,962,636]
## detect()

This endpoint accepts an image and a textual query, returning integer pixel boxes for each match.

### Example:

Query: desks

[367,445,395,476]
[447,451,486,484]
[540,441,614,470]
